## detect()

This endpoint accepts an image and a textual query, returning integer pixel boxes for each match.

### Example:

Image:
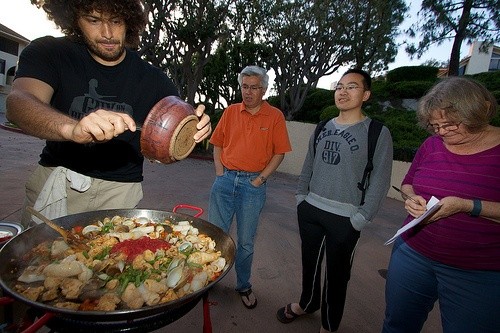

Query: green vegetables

[41,220,206,292]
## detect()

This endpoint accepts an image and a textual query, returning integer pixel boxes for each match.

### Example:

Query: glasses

[425,120,462,133]
[240,84,263,91]
[334,85,363,91]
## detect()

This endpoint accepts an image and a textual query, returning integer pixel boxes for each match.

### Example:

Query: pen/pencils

[392,185,428,211]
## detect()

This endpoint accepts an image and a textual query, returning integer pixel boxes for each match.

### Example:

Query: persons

[210,66,293,310]
[276,69,392,333]
[6,0,212,231]
[382,76,500,333]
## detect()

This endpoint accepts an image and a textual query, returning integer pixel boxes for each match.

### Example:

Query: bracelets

[469,199,482,217]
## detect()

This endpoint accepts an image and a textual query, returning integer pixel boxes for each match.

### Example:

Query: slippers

[240,283,257,309]
[276,303,314,324]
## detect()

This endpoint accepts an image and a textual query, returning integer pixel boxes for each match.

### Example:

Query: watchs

[258,174,267,183]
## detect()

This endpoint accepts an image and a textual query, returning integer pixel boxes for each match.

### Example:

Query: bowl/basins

[0,221,24,249]
[140,95,200,165]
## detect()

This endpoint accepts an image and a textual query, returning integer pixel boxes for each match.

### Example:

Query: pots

[0,204,236,333]
